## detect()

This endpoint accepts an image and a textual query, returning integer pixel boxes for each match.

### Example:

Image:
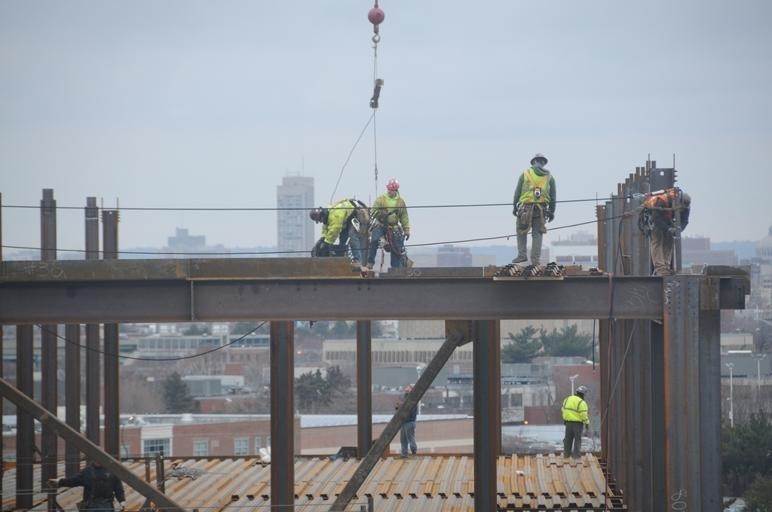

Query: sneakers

[511,256,527,264]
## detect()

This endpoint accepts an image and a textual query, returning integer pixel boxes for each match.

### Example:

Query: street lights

[569,373,579,396]
[725,363,735,428]
[415,364,422,414]
[736,327,746,346]
[755,328,762,353]
[750,353,767,394]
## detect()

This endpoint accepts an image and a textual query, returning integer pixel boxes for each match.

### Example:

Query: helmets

[309,208,323,224]
[576,385,588,395]
[387,178,399,192]
[530,153,548,167]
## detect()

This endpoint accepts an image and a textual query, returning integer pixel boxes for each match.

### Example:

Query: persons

[310,199,372,267]
[561,385,590,458]
[47,445,126,511]
[366,179,409,270]
[642,191,691,274]
[512,153,556,264]
[394,378,418,459]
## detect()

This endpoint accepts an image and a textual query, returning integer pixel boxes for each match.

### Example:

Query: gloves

[120,501,128,511]
[47,476,60,489]
